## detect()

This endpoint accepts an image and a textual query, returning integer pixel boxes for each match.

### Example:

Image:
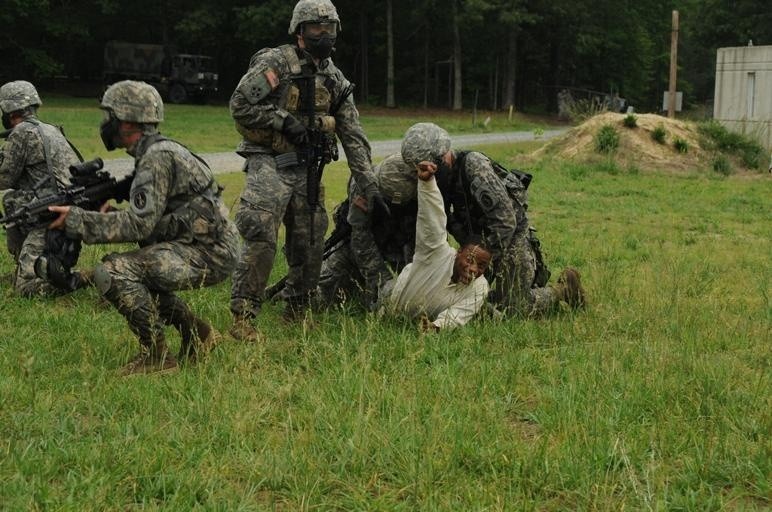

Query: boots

[545,268,588,313]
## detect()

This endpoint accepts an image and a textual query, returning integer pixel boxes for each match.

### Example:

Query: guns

[275,74,325,247]
[0,128,13,140]
[265,198,390,302]
[510,168,533,190]
[0,157,133,229]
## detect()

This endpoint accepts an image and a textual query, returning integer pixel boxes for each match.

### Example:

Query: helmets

[379,153,419,204]
[0,80,41,116]
[99,78,164,125]
[288,0,342,35]
[400,121,451,163]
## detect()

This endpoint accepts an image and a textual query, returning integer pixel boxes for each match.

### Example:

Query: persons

[47,81,240,378]
[555,86,624,121]
[1,79,96,299]
[272,122,586,331]
[228,0,390,342]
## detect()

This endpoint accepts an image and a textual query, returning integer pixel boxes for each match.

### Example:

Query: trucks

[98,42,219,104]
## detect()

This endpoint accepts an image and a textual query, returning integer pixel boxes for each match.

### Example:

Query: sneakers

[178,321,221,363]
[278,305,314,338]
[116,340,177,379]
[224,315,268,343]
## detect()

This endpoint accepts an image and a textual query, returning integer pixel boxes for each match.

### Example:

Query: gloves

[283,124,310,145]
[364,184,391,216]
